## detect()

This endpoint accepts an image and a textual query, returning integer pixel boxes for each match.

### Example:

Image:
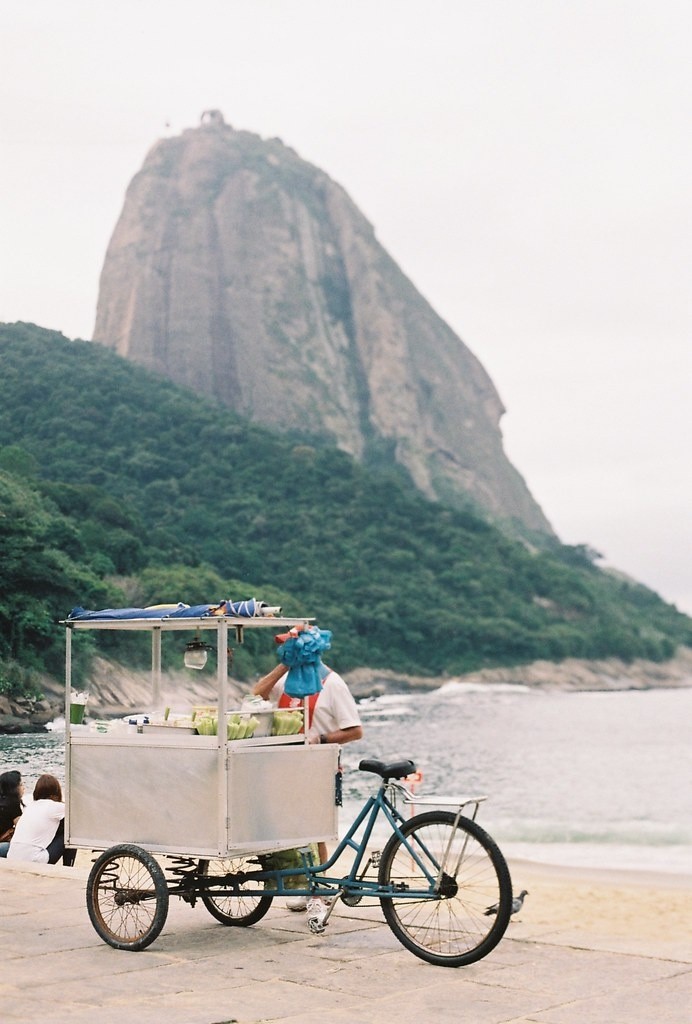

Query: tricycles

[68,616,514,965]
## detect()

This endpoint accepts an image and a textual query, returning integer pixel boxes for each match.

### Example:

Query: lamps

[183,637,217,670]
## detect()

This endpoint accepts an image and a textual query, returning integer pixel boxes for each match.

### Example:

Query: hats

[275,624,314,644]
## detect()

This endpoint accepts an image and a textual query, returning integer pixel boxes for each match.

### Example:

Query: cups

[70,703,86,725]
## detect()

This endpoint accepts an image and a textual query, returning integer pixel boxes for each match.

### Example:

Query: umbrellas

[68,598,283,620]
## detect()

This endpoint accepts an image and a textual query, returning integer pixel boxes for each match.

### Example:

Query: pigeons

[483,889,530,924]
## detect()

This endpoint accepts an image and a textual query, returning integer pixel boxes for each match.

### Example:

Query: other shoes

[308,899,327,921]
[287,896,305,911]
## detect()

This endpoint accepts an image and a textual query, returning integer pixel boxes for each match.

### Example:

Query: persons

[7,774,77,867]
[0,770,25,856]
[252,625,363,922]
